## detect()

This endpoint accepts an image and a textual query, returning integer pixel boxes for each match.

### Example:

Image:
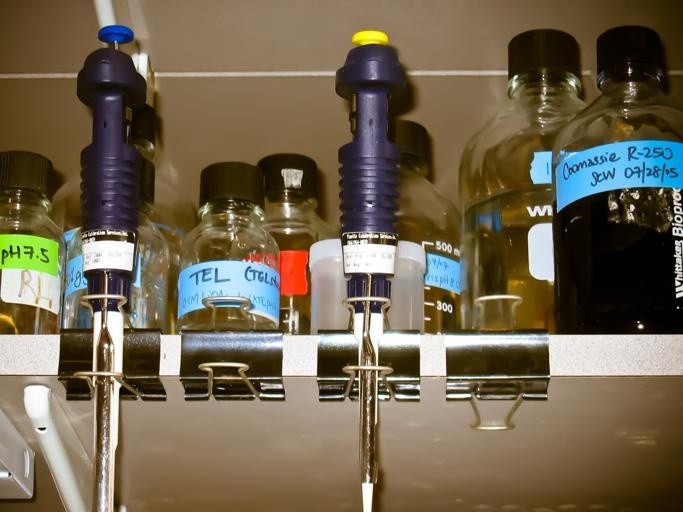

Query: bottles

[60,154,172,336]
[253,152,338,336]
[166,162,282,336]
[50,101,199,337]
[0,150,69,336]
[457,28,589,333]
[551,24,683,336]
[379,115,461,335]
